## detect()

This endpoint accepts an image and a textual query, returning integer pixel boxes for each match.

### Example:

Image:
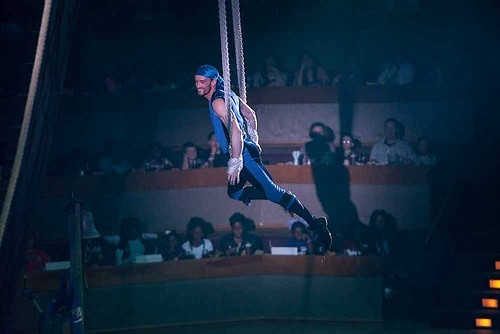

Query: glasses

[342,141,351,143]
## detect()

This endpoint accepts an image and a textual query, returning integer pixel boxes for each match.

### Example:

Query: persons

[194,66,332,250]
[292,221,310,247]
[115,216,146,262]
[181,142,207,169]
[206,131,231,168]
[337,133,362,167]
[370,118,412,166]
[378,47,418,86]
[293,50,332,87]
[369,209,399,305]
[140,143,178,171]
[246,50,287,90]
[333,55,365,84]
[181,217,216,260]
[415,137,438,167]
[301,122,336,164]
[221,212,264,257]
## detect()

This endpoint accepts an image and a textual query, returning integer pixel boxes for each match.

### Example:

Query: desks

[0,252,384,326]
[0,88,445,153]
[1,167,438,234]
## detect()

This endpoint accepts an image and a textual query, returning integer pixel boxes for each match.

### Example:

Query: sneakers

[288,190,294,217]
[309,217,332,249]
